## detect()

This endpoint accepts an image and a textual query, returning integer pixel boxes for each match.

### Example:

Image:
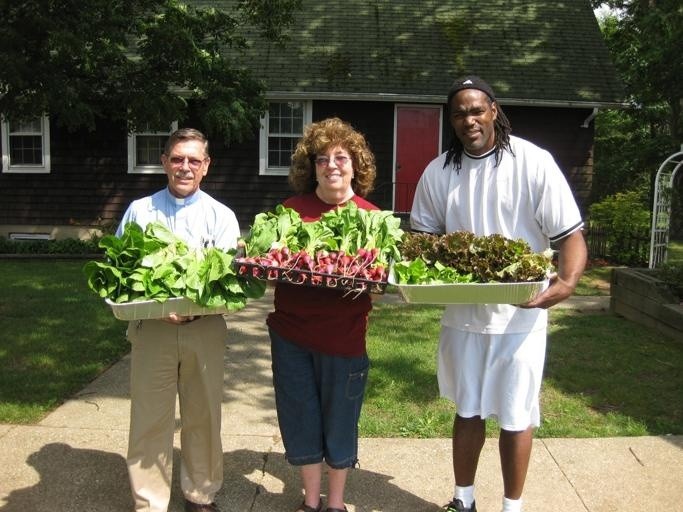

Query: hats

[448,75,495,103]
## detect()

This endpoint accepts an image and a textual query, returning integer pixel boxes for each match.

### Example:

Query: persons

[257,115,397,510]
[104,126,246,512]
[406,74,589,511]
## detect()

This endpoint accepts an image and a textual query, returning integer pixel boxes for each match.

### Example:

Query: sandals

[295,498,348,512]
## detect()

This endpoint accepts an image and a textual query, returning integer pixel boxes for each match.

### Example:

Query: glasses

[315,156,352,167]
[170,157,205,170]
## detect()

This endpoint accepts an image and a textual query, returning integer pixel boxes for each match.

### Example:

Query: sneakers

[443,498,477,512]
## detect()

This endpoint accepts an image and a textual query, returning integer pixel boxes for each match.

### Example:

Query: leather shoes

[186,501,220,512]
